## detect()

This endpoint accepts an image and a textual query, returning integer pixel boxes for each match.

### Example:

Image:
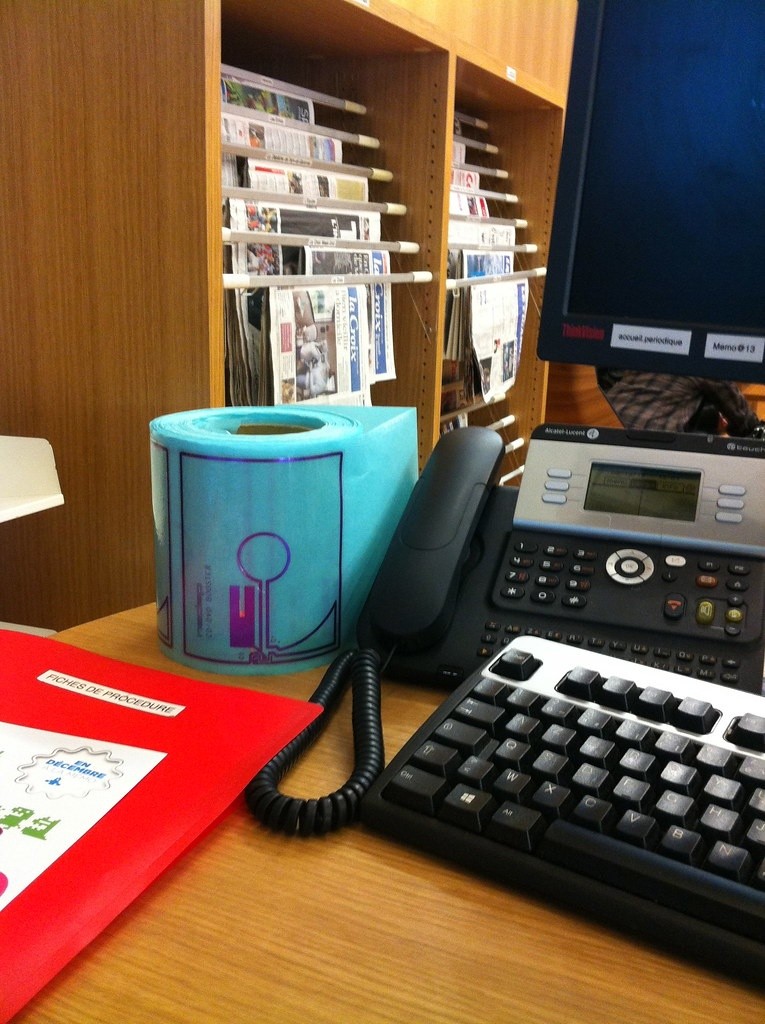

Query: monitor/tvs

[537,0,765,379]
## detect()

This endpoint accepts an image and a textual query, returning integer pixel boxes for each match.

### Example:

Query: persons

[564,364,753,437]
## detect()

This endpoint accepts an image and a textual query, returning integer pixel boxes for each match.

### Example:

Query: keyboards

[358,634,765,983]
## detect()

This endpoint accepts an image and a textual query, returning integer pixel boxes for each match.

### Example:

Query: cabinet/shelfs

[0,0,566,635]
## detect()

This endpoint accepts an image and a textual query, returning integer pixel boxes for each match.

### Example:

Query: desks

[0,603,765,1024]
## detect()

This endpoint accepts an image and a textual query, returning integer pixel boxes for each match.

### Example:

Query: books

[0,625,326,1024]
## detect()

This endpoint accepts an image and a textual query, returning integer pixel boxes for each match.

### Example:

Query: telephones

[356,422,764,691]
